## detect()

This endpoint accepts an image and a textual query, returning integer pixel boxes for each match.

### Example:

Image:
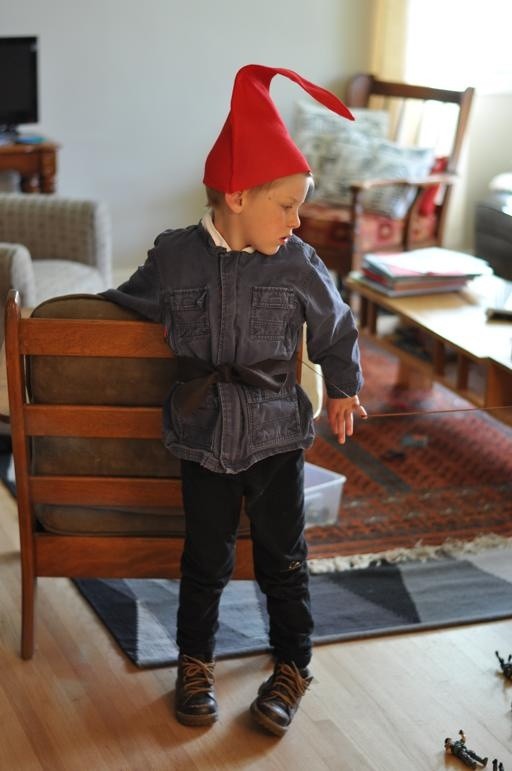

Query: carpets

[71,547,512,668]
[267,335,512,575]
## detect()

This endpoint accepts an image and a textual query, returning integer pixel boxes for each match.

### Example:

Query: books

[359,246,493,299]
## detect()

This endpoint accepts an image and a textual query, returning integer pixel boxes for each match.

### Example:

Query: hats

[202,64,355,193]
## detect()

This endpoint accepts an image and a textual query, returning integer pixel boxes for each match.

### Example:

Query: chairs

[6,288,303,661]
[290,74,475,332]
[0,196,113,413]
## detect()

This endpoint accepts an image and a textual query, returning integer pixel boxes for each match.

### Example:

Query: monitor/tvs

[0,36,38,134]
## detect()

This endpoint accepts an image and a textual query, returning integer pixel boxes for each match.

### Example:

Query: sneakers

[175,652,218,727]
[248,662,313,736]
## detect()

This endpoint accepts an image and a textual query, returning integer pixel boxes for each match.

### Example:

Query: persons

[96,166,371,736]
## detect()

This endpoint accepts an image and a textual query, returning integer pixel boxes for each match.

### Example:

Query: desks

[343,270,511,427]
[0,134,61,193]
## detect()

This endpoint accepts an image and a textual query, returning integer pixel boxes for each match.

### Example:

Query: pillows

[25,295,252,539]
[294,101,449,219]
